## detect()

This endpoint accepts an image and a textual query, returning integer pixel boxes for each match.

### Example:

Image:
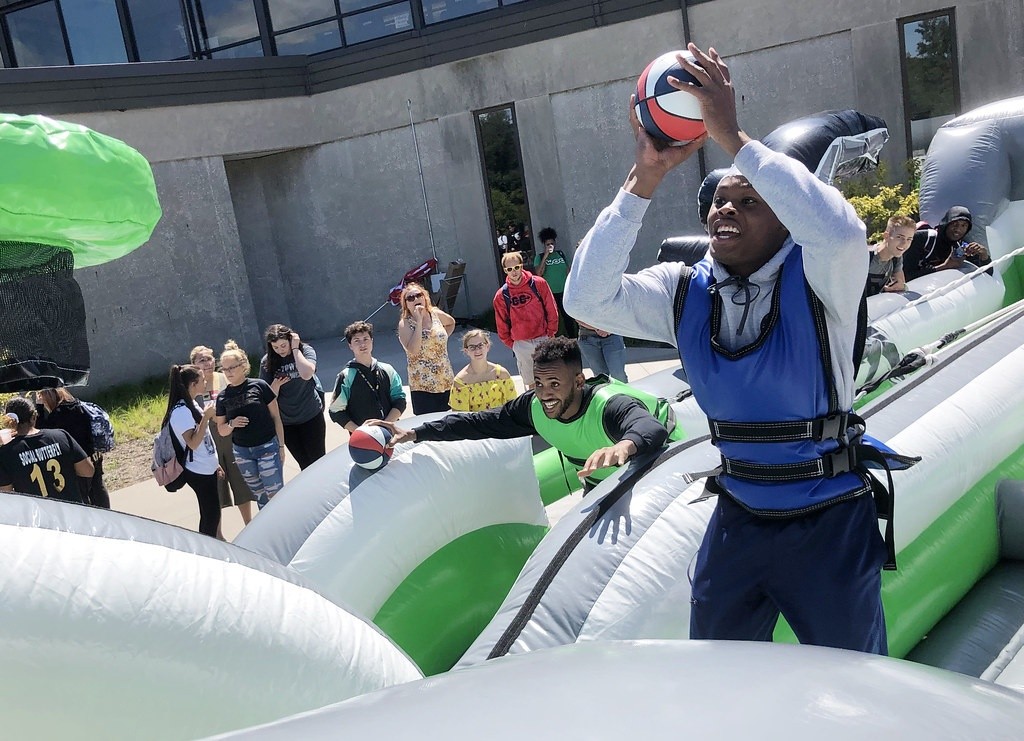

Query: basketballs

[348,423,395,471]
[633,48,708,146]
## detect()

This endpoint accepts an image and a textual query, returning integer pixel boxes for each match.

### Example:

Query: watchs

[227,419,234,429]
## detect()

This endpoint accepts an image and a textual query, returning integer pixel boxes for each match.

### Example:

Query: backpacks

[61,400,116,452]
[151,403,196,493]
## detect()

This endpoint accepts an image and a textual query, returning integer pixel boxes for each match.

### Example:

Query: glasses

[504,264,522,273]
[405,292,423,302]
[218,363,242,373]
[546,243,553,246]
[467,341,486,351]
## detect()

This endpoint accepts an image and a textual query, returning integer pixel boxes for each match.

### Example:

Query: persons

[0,387,110,509]
[398,283,455,416]
[329,321,407,436]
[534,227,580,338]
[362,336,685,497]
[576,239,628,384]
[448,329,517,412]
[496,222,531,260]
[161,340,285,542]
[863,206,916,299]
[903,206,993,284]
[563,42,922,656]
[258,324,326,471]
[493,252,558,389]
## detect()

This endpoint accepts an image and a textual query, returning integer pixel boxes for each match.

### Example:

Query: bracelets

[291,348,299,350]
[279,446,284,448]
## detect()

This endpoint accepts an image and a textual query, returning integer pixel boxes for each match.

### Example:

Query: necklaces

[470,361,488,382]
[878,246,891,276]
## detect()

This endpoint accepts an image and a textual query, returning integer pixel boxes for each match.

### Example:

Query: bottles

[953,242,969,258]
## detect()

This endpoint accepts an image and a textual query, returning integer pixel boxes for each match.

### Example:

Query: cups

[548,245,554,253]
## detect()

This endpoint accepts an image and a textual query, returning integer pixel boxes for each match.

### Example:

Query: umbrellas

[341,258,438,340]
